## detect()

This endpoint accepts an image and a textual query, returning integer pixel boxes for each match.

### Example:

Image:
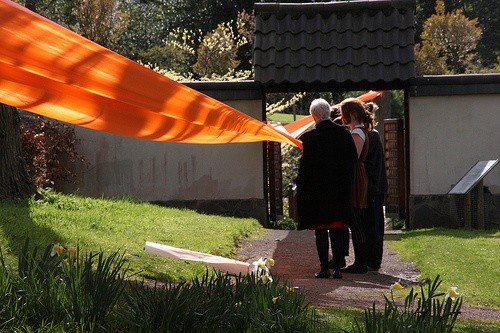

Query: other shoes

[340,263,368,274]
[320,258,346,268]
[369,265,379,270]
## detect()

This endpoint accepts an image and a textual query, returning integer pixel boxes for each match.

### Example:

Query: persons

[343,99,387,273]
[295,98,359,280]
[320,106,350,269]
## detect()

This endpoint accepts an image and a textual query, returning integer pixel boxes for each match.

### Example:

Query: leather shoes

[315,270,331,279]
[333,270,342,279]
